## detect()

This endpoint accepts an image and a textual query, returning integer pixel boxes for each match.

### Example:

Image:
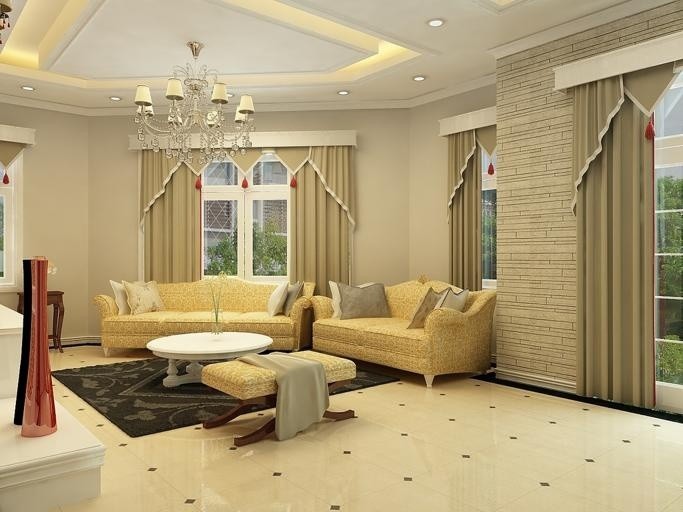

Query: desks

[16,291,65,353]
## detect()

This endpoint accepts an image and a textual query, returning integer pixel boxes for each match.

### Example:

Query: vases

[210,296,225,343]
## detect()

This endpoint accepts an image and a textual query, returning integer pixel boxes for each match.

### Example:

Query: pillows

[108,278,166,316]
[406,286,471,329]
[266,280,306,317]
[328,281,393,321]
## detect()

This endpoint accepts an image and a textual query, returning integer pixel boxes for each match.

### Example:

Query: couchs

[93,275,315,361]
[310,279,497,388]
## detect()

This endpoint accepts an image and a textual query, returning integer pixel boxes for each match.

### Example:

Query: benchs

[202,350,357,448]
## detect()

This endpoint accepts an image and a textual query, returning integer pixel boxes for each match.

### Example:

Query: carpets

[50,355,403,437]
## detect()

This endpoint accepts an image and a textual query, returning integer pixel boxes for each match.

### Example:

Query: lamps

[131,42,257,164]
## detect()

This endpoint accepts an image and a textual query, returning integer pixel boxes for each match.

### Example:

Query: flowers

[201,271,229,336]
